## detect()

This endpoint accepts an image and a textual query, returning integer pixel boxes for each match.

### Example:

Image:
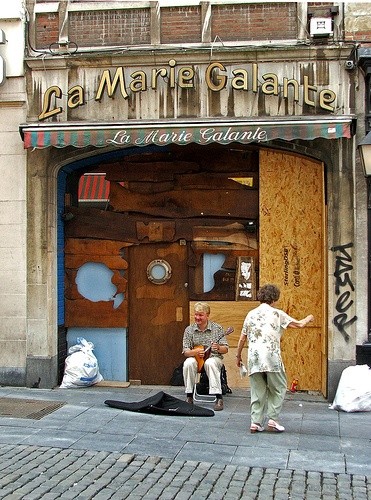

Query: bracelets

[236,355,242,358]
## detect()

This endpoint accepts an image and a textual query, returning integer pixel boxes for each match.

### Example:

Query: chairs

[192,372,218,403]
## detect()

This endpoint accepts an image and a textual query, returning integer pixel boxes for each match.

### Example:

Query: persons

[181,302,229,411]
[235,284,314,432]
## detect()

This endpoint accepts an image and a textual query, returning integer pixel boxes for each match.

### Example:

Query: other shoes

[184,397,193,405]
[214,398,224,411]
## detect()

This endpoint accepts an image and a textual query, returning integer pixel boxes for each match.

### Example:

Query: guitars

[190,326,234,373]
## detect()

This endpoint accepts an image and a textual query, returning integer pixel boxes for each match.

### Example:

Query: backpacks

[197,366,233,394]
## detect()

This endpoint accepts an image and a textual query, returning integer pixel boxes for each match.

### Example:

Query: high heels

[249,423,264,433]
[268,419,285,432]
